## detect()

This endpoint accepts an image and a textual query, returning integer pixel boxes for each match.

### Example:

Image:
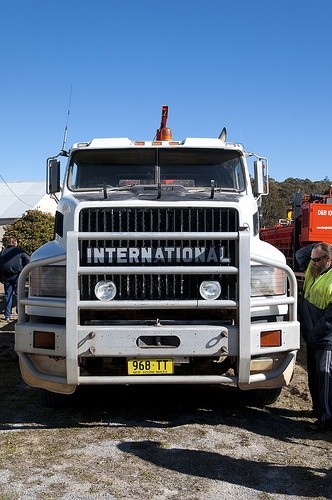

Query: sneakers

[308,409,321,416]
[308,420,331,433]
[5,317,11,323]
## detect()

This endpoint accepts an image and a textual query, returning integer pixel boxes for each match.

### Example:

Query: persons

[0,237,30,321]
[296,242,332,432]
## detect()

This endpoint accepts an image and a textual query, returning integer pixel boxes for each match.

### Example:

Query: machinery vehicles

[258,184,332,291]
[13,105,302,408]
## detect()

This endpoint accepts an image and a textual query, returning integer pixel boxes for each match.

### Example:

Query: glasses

[310,256,329,262]
